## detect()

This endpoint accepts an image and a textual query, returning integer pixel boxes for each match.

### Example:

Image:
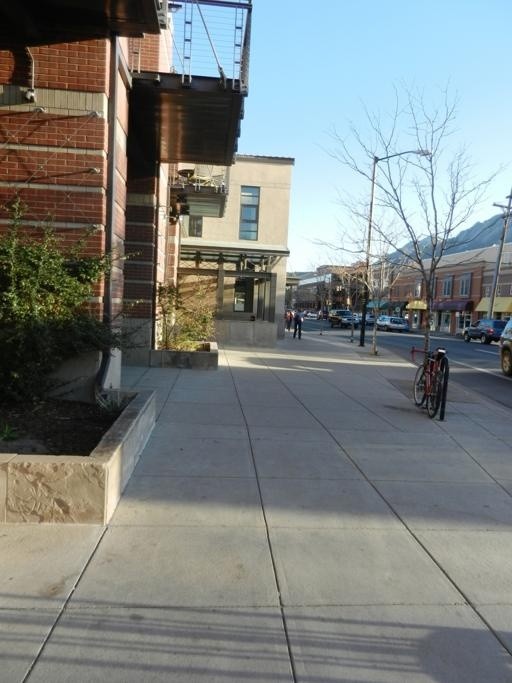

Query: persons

[286,308,294,331]
[292,307,305,340]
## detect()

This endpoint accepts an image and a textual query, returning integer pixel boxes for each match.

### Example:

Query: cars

[462,319,508,344]
[317,310,410,332]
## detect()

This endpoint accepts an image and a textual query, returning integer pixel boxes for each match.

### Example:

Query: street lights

[360,150,432,347]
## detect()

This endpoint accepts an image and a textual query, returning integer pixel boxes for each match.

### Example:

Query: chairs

[169,164,214,186]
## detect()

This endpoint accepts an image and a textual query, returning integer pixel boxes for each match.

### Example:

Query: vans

[500,319,512,376]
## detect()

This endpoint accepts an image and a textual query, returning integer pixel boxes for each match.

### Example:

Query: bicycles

[411,346,449,420]
